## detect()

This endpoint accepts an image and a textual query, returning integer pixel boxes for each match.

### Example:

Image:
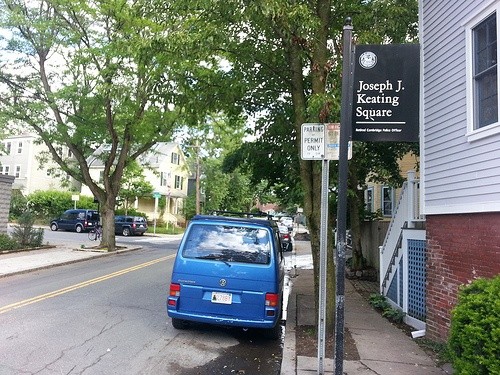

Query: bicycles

[87,222,102,241]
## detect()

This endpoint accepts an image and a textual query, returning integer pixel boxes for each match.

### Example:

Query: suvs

[114,215,149,236]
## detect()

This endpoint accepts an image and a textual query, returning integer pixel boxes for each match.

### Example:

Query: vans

[165,209,293,338]
[50,208,101,233]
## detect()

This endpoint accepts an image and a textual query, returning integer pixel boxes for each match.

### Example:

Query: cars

[273,216,294,251]
